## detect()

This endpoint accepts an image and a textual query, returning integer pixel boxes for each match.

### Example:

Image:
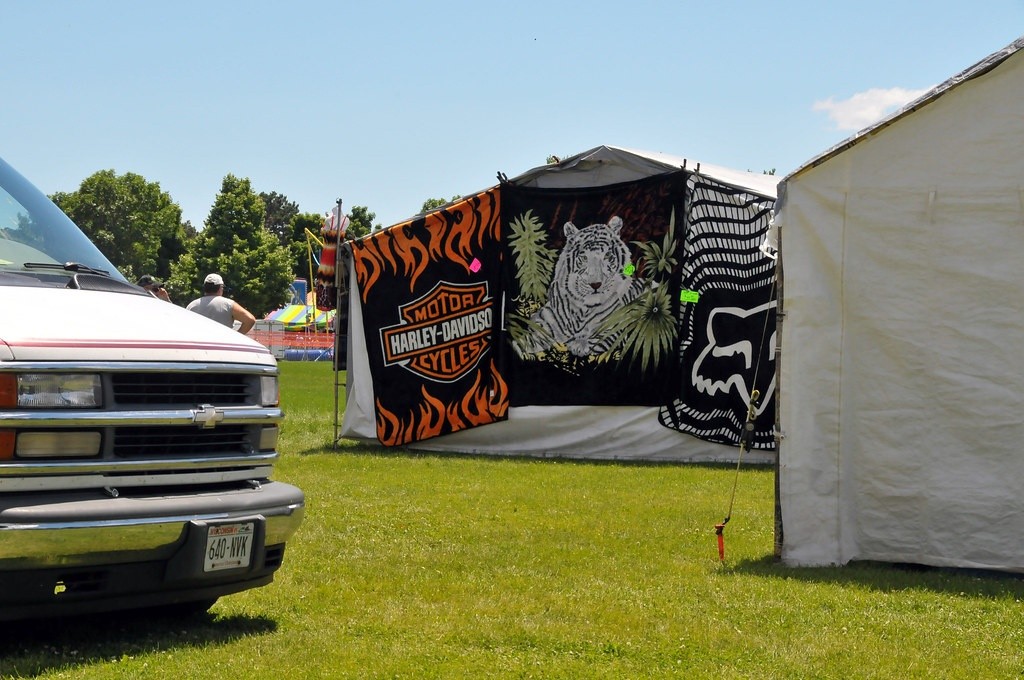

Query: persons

[137,275,171,304]
[185,273,255,334]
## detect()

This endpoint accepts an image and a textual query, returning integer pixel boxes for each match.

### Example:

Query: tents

[331,145,786,468]
[767,37,1024,573]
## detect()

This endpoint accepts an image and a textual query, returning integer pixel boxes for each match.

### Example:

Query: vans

[0,152,305,622]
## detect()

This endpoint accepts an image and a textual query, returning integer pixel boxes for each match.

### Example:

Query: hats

[204,273,225,285]
[137,275,161,288]
